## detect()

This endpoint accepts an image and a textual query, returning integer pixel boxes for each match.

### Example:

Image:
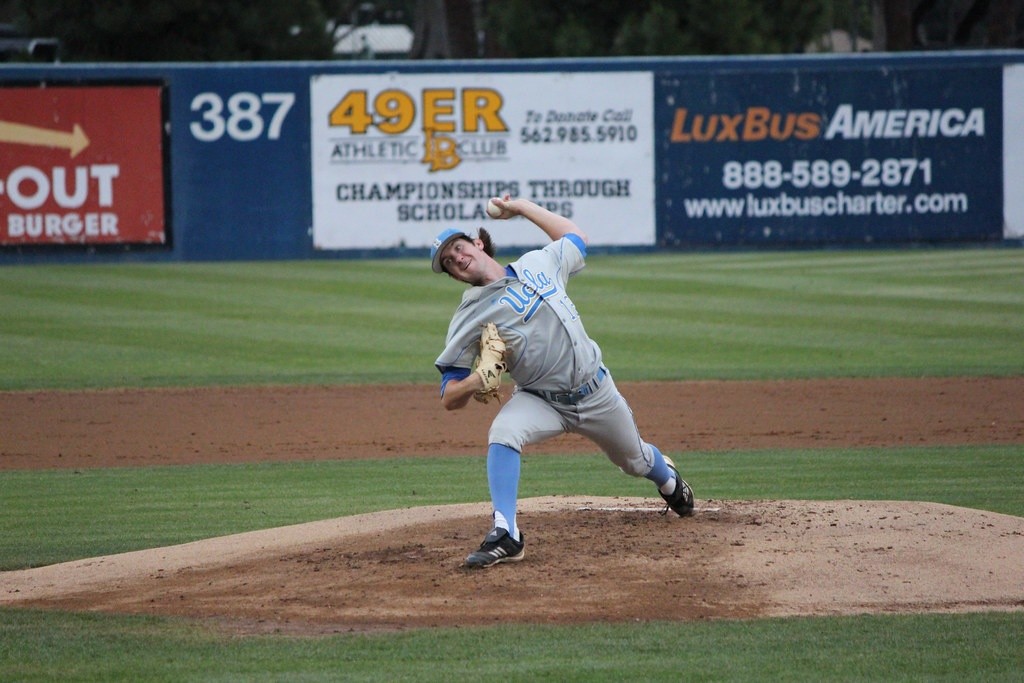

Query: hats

[429,227,465,274]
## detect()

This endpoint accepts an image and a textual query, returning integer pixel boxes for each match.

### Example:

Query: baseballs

[487,197,504,218]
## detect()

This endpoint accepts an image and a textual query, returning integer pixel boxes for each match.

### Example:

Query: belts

[516,364,607,404]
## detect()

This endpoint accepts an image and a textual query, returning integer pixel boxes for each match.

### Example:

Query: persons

[432,194,693,569]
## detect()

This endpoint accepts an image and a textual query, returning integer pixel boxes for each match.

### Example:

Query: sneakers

[657,454,695,518]
[463,526,526,569]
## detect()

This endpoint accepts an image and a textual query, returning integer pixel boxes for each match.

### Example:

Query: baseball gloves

[474,322,507,405]
